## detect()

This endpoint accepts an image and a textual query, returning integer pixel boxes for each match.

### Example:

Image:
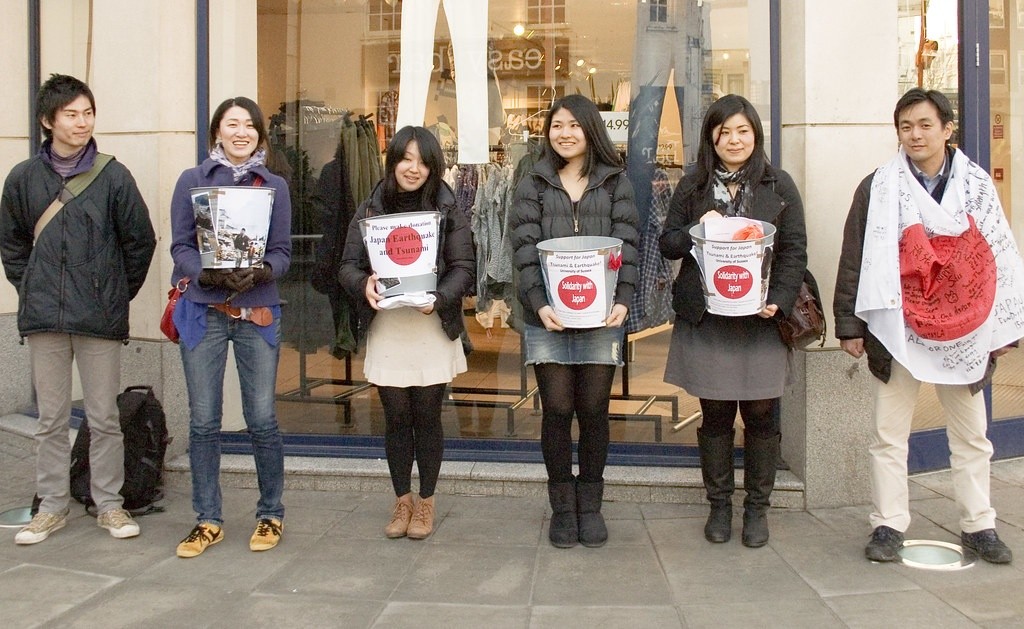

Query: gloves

[200,264,272,302]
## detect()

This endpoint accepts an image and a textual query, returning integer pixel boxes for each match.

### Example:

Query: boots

[549,475,579,547]
[697,427,736,542]
[577,476,608,546]
[743,428,782,547]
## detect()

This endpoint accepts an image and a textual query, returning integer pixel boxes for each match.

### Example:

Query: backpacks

[70,386,169,505]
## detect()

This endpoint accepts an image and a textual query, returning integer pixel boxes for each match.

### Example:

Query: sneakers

[865,526,904,560]
[250,518,283,551]
[387,490,414,537]
[15,513,66,544]
[177,523,224,557]
[407,495,434,538]
[97,509,140,537]
[961,529,1012,563]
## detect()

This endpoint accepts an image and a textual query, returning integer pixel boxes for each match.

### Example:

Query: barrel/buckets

[534,235,624,330]
[357,210,444,297]
[689,218,776,317]
[188,185,276,269]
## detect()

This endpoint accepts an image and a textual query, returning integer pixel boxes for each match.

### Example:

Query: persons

[233,229,255,269]
[659,94,808,547]
[833,86,1024,562]
[338,126,476,539]
[0,73,157,544]
[169,97,291,557]
[511,95,640,547]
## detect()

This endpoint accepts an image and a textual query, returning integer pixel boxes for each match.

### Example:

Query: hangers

[302,105,348,125]
[509,87,557,136]
[444,141,630,167]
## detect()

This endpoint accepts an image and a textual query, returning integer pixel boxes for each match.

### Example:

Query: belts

[208,303,273,326]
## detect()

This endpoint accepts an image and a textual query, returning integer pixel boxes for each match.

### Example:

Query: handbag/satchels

[776,282,825,350]
[160,288,181,343]
